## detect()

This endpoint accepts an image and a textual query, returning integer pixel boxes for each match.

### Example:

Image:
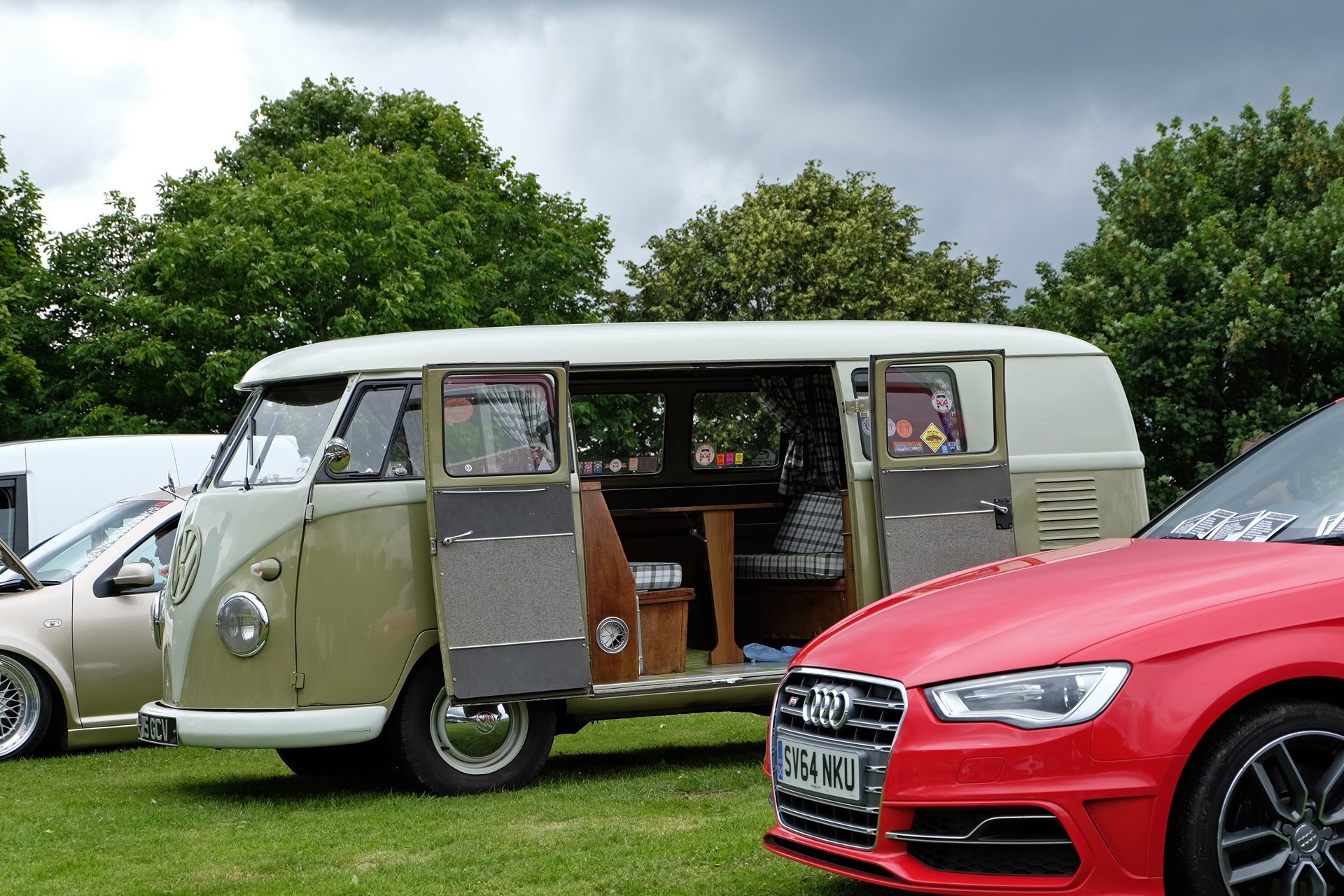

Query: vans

[140,320,1153,800]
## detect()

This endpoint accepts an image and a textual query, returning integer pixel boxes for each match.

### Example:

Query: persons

[139,519,180,568]
[1240,432,1306,509]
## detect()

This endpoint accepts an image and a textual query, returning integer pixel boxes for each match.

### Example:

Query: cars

[759,394,1344,896]
[0,487,192,762]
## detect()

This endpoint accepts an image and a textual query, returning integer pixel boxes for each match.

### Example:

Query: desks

[645,502,774,665]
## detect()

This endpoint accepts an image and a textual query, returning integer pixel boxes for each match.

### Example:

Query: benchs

[734,490,851,644]
[579,482,695,683]
